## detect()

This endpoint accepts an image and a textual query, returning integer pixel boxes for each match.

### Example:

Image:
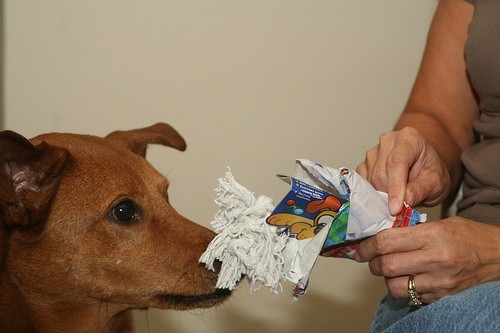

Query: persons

[353,0,500,333]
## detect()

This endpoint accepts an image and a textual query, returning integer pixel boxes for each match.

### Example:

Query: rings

[406,275,423,307]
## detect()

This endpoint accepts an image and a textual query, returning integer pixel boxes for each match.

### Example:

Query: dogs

[0,121,247,333]
